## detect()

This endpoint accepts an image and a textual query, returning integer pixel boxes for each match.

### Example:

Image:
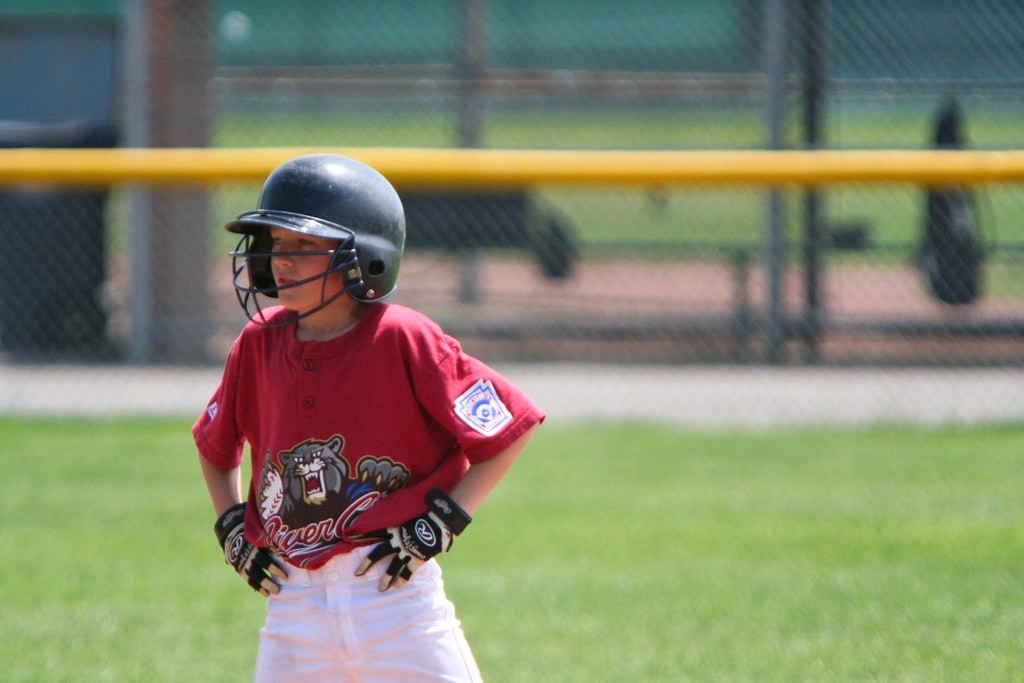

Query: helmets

[225,156,405,304]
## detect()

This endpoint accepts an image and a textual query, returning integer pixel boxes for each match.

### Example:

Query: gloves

[351,489,471,592]
[213,503,287,596]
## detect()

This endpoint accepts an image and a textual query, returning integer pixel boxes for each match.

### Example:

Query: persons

[192,154,546,683]
